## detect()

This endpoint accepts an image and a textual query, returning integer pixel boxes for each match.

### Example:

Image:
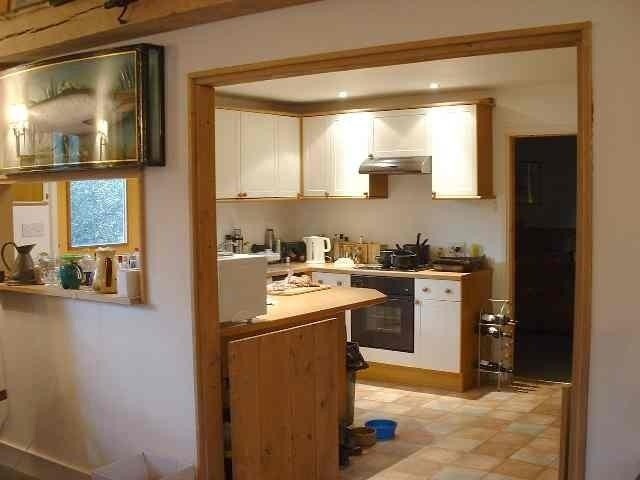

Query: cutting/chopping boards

[267,284,332,296]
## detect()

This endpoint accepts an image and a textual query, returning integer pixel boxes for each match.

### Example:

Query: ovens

[350,274,415,353]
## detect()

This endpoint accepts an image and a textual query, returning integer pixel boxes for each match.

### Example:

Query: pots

[375,245,430,269]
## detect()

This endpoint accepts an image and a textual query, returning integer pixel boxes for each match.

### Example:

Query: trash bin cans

[344,342,369,426]
[92,449,193,480]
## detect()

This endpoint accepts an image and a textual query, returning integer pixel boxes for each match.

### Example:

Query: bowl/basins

[365,420,396,440]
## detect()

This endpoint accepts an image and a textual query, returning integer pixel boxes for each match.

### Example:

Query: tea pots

[0,241,37,285]
[91,248,120,294]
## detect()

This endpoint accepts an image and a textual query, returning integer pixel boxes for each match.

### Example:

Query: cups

[117,268,140,298]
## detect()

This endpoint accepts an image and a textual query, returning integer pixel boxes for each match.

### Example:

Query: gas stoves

[358,264,434,271]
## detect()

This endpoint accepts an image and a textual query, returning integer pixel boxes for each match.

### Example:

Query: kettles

[303,236,331,265]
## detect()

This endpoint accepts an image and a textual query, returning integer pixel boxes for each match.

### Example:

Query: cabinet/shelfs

[277,104,492,198]
[310,270,351,343]
[214,107,276,200]
[413,279,491,373]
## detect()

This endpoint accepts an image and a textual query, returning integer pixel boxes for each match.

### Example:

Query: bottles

[59,255,84,290]
[266,230,274,249]
[360,236,364,244]
[226,228,243,254]
[475,313,519,374]
[129,248,140,268]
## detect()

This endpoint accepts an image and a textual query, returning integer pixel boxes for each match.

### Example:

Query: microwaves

[218,252,267,323]
